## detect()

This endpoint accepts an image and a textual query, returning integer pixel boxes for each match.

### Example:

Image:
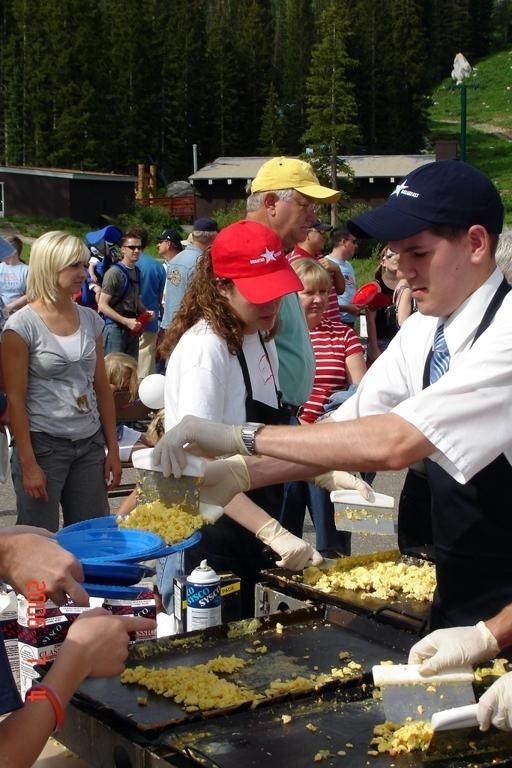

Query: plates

[51,511,205,599]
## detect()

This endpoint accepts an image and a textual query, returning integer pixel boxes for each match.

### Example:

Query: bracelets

[241,413,268,458]
[23,681,67,737]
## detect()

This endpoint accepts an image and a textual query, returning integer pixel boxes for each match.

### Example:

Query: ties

[427,324,450,385]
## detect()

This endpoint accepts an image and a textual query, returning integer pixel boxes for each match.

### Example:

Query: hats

[210,220,305,305]
[250,158,342,204]
[310,218,333,232]
[193,217,217,231]
[155,230,183,246]
[346,158,503,241]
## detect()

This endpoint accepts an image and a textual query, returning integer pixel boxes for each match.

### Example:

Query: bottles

[130,310,152,337]
[181,558,225,633]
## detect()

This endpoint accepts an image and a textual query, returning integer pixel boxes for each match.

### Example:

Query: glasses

[285,192,321,212]
[122,244,142,250]
[336,238,357,245]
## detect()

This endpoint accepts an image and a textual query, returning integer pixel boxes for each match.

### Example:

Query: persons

[489,218,512,289]
[0,607,163,768]
[241,152,316,539]
[0,520,95,608]
[400,592,512,734]
[1,211,419,640]
[152,157,511,671]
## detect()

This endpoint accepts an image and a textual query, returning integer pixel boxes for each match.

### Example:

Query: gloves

[315,470,375,500]
[152,415,254,524]
[477,670,512,733]
[255,518,324,572]
[407,621,501,675]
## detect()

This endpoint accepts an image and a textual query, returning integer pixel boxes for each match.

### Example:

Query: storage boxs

[104,424,142,462]
